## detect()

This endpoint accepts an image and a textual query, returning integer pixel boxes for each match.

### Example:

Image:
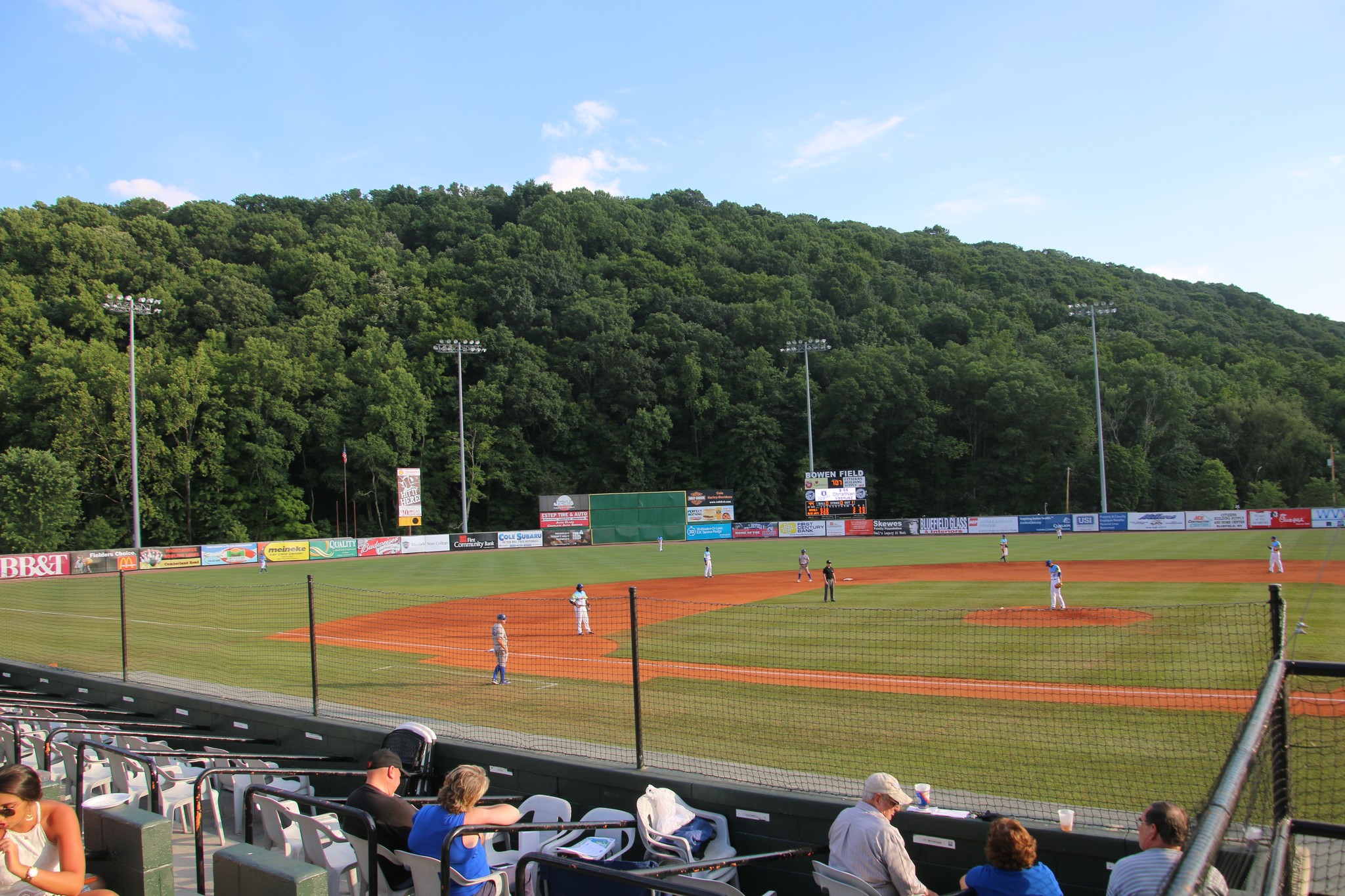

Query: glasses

[873,793,900,806]
[1136,819,1158,829]
[0,800,25,816]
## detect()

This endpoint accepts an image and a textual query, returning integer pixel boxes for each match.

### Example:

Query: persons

[1045,560,1065,610]
[823,560,837,603]
[1055,523,1062,540]
[903,518,920,535]
[75,555,92,574]
[0,764,120,896]
[491,614,512,684]
[259,553,268,573]
[704,547,713,578]
[1000,535,1009,563]
[343,748,420,891]
[569,583,595,635]
[408,765,536,896]
[1106,801,1230,896]
[1267,536,1284,573]
[657,536,663,551]
[960,818,1064,896]
[828,773,939,896]
[797,549,812,582]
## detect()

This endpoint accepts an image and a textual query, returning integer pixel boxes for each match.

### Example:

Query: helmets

[801,549,806,554]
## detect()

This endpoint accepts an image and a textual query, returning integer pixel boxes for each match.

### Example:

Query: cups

[914,783,930,811]
[1242,826,1262,852]
[1057,809,1074,831]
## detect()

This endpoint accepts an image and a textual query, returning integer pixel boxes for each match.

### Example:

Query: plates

[81,792,131,809]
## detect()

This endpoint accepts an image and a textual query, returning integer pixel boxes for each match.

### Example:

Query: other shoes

[999,558,1008,563]
[1049,606,1066,609]
[824,599,835,603]
[1267,571,1281,574]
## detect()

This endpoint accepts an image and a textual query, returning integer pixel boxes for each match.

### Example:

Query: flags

[342,445,347,463]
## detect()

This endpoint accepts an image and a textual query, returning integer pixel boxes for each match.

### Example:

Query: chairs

[0,700,881,896]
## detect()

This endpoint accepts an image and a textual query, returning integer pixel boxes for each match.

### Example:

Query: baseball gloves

[1054,583,1062,588]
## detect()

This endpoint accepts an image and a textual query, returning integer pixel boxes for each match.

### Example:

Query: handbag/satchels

[646,783,696,843]
[658,816,716,861]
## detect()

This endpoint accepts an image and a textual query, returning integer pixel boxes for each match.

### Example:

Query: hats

[1270,536,1277,539]
[367,748,411,777]
[1057,523,1059,524]
[497,614,508,619]
[1045,560,1052,567]
[1002,534,1006,537]
[864,772,914,805]
[706,546,709,549]
[577,583,584,588]
[826,560,831,564]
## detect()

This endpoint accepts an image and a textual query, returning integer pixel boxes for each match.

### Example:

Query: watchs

[21,866,39,882]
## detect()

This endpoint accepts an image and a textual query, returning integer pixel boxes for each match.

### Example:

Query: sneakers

[578,633,585,635]
[499,679,511,684]
[586,632,595,634]
[705,576,714,578]
[491,679,499,685]
[797,579,801,582]
[808,579,813,582]
